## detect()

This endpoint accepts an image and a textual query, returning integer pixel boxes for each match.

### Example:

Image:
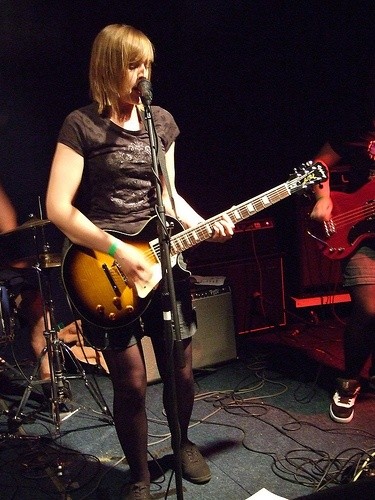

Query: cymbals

[7,251,62,269]
[0,217,53,238]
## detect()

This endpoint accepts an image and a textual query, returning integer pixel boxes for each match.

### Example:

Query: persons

[47,23,235,499]
[0,191,65,411]
[312,102,375,425]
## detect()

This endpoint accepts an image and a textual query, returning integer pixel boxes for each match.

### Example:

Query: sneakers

[328,378,362,422]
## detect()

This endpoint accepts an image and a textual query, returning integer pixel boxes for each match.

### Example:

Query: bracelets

[107,239,121,257]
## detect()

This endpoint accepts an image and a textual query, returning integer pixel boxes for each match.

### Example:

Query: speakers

[140,284,238,383]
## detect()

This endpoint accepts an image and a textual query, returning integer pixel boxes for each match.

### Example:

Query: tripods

[8,226,116,432]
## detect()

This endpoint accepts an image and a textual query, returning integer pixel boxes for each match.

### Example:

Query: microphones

[137,79,152,105]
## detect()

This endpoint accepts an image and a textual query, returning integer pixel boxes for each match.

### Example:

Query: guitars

[305,177,375,261]
[60,157,330,329]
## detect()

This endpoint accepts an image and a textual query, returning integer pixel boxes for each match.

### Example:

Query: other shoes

[126,481,150,500]
[173,444,212,482]
